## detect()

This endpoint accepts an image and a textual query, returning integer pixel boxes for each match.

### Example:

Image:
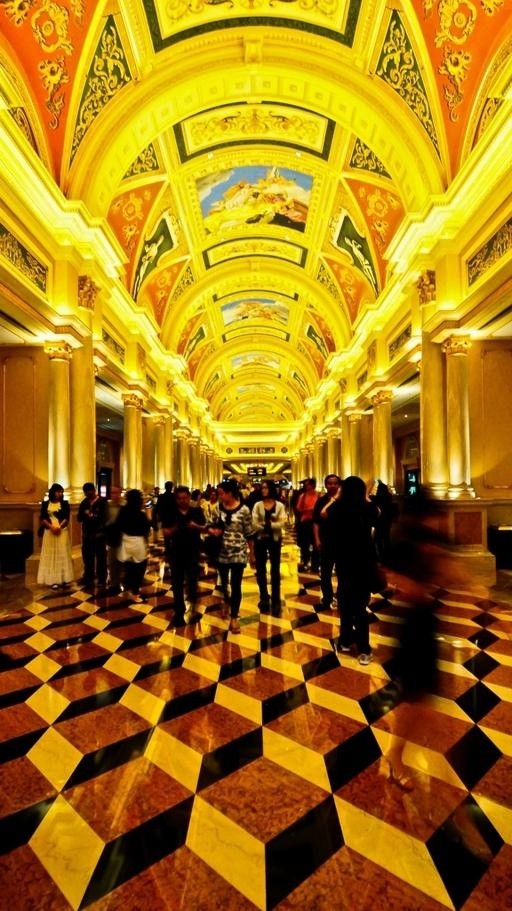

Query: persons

[77,483,149,603]
[37,483,74,589]
[374,486,464,799]
[319,474,376,665]
[146,479,288,627]
[291,475,391,606]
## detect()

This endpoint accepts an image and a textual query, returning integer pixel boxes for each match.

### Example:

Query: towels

[487,525,512,569]
[0,531,34,575]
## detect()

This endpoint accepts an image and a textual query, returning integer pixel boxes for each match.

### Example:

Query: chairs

[359,654,374,664]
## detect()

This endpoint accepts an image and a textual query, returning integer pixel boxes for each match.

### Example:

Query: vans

[382,758,414,791]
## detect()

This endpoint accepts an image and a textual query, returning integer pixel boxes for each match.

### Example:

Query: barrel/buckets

[230,622,240,634]
